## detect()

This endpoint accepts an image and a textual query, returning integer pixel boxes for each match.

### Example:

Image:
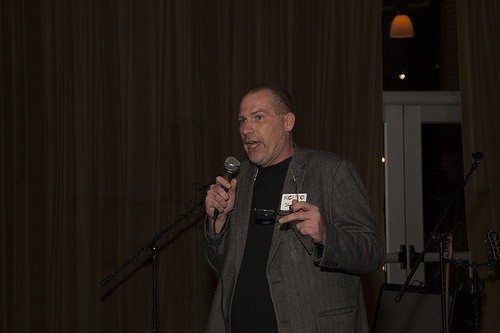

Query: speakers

[477,263,500,333]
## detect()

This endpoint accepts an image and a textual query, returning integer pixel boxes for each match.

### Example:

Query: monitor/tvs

[372,283,455,333]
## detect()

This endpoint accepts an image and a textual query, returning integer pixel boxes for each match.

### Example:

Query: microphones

[213,156,241,220]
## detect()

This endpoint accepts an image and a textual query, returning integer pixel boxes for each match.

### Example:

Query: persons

[203,85,384,333]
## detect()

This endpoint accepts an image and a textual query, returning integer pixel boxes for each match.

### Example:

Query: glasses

[253,167,297,216]
